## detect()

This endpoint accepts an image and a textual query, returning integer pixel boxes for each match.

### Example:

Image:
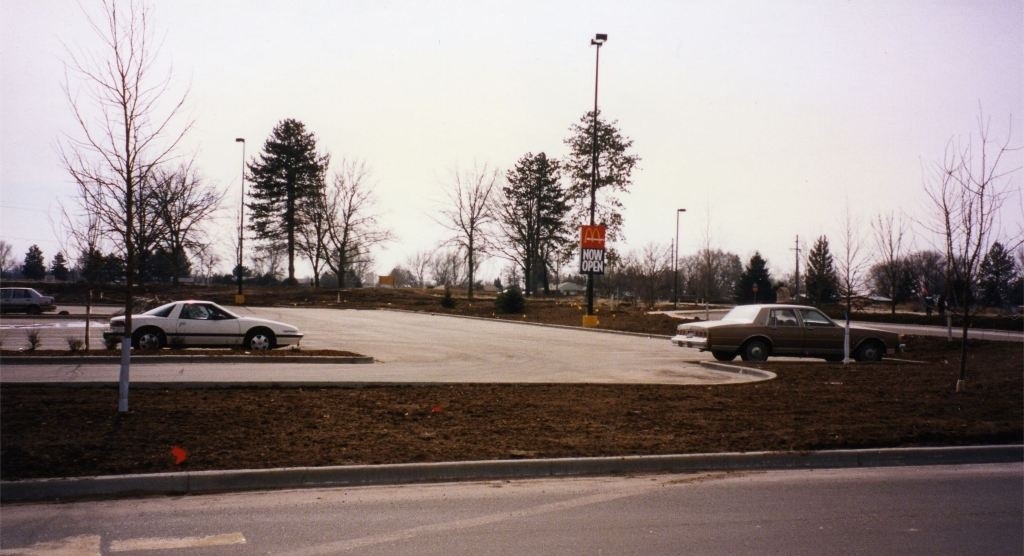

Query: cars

[0,288,56,316]
[673,304,900,364]
[101,300,304,352]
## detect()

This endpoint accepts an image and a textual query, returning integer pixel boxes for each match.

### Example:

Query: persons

[937,299,945,320]
[925,299,934,320]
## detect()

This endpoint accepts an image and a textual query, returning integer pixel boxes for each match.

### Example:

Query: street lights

[582,34,607,329]
[675,209,685,305]
[234,138,245,305]
